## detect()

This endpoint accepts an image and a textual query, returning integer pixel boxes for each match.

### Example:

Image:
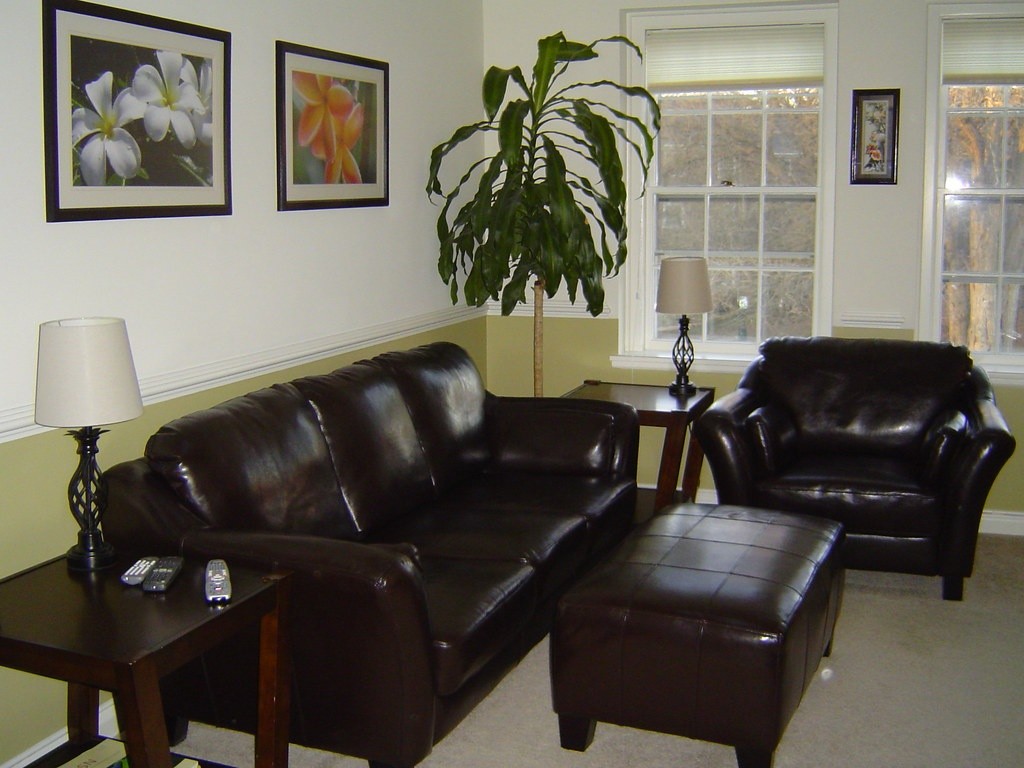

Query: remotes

[121,556,159,586]
[203,559,231,603]
[142,556,183,592]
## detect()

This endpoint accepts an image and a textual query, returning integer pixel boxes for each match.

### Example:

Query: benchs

[550,501,845,768]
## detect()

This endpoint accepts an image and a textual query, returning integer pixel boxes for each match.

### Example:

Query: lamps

[35,318,143,572]
[655,257,714,395]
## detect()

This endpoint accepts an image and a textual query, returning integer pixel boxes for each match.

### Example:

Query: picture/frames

[275,40,390,211]
[42,0,232,223]
[850,89,900,185]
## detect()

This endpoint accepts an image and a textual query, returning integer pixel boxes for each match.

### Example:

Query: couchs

[693,336,1016,602]
[97,341,640,768]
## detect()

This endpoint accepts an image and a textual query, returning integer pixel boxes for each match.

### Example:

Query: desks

[0,551,297,768]
[560,379,714,510]
